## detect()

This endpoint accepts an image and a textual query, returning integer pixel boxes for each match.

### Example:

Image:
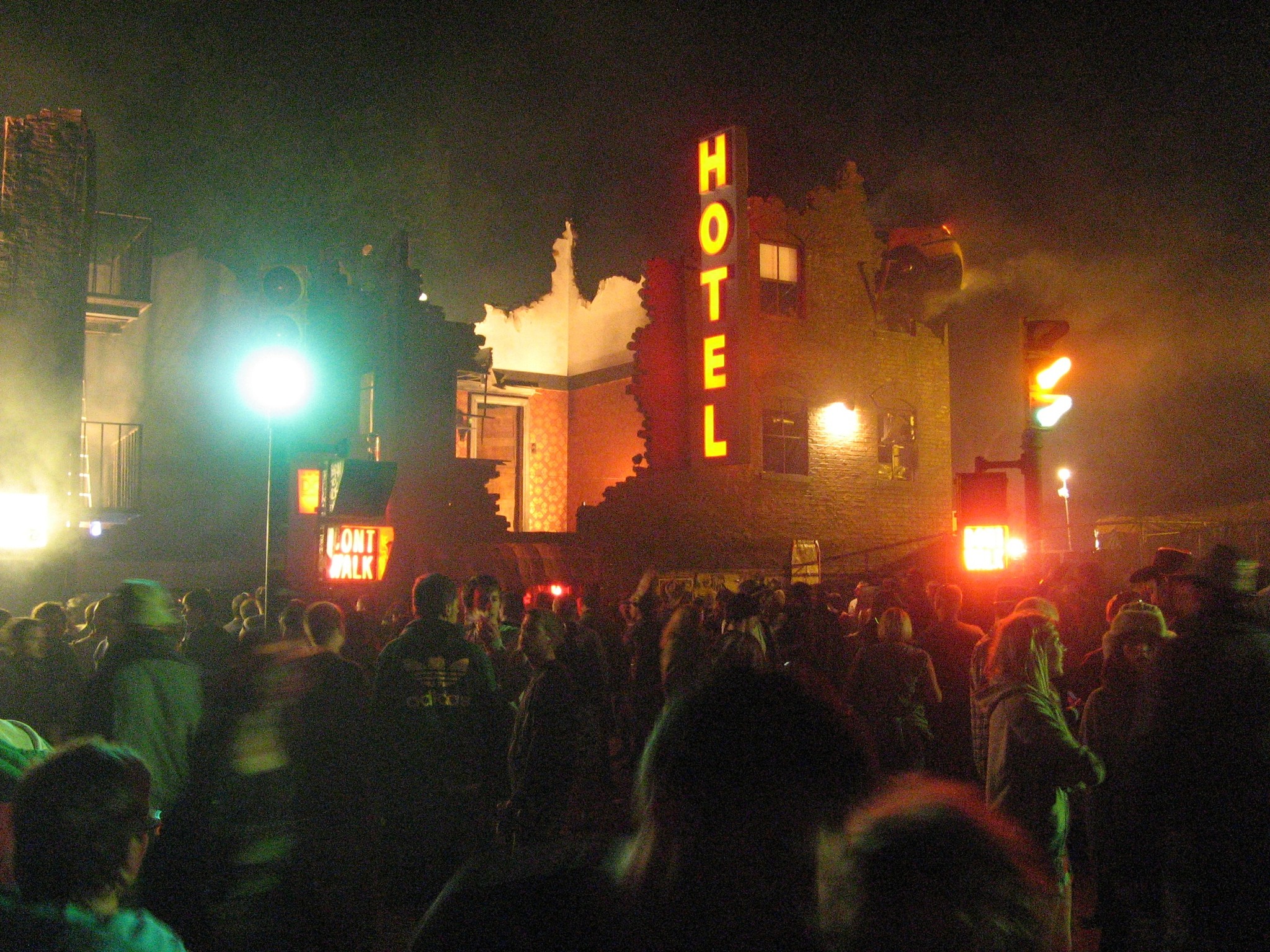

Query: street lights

[1055,467,1073,553]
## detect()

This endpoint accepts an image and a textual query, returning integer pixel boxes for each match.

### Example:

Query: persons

[0,542,1270,952]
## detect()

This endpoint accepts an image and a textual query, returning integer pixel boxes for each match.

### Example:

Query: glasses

[127,808,164,836]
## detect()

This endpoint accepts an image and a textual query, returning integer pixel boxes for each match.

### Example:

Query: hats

[1128,547,1199,586]
[118,581,178,629]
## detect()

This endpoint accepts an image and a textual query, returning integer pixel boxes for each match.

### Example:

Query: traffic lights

[952,471,1011,574]
[1022,317,1074,431]
[248,260,312,425]
